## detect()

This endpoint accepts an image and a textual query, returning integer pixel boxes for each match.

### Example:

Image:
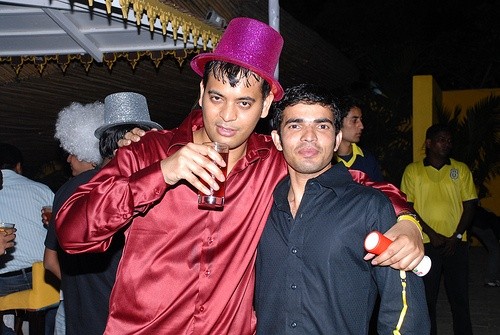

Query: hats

[190,17,284,102]
[94,92,163,140]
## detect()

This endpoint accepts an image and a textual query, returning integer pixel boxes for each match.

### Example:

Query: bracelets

[396,211,424,240]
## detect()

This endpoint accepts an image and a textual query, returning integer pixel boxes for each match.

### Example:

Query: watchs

[454,232,463,240]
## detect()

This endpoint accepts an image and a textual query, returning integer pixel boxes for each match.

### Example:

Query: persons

[112,82,432,335]
[400,124,479,335]
[0,92,165,335]
[330,95,384,181]
[54,16,424,335]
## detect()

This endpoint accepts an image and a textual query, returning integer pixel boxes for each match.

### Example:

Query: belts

[0,267,32,277]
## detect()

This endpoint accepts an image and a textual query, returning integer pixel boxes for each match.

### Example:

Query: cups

[0,223,15,254]
[197,142,229,211]
[42,206,53,221]
